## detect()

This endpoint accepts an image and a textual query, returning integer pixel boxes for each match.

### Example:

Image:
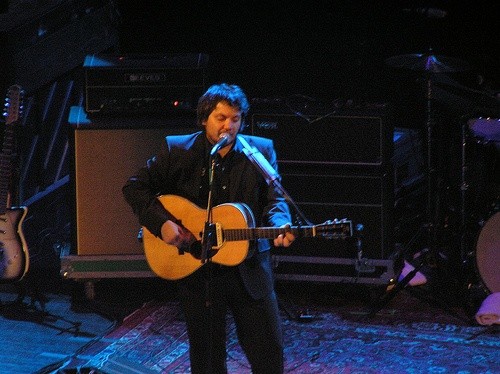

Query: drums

[464,203,500,301]
[454,111,500,146]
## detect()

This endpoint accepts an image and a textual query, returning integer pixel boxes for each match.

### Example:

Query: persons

[122,83,296,374]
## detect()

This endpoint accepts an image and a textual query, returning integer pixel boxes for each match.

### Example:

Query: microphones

[210,132,232,158]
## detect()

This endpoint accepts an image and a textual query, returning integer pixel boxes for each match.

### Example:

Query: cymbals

[384,54,461,73]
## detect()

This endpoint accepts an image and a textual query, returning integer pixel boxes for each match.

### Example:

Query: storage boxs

[60,105,436,286]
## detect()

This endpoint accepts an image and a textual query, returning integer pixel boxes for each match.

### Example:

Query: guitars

[0,85,31,282]
[143,193,354,282]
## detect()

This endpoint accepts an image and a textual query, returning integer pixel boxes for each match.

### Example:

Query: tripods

[369,46,500,318]
